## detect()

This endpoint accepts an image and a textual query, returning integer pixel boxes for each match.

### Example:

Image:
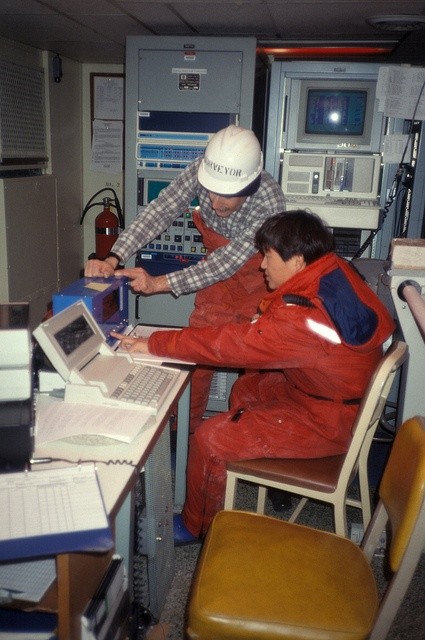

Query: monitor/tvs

[294,78,377,152]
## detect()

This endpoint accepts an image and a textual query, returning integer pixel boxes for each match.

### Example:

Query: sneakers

[268,488,293,512]
[173,513,197,545]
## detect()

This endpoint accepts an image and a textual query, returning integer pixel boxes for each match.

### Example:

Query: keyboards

[109,364,181,415]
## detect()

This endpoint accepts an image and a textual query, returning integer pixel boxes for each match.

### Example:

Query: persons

[84,124,293,513]
[110,210,396,548]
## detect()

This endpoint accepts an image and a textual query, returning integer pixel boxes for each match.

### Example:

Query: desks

[0,362,195,640]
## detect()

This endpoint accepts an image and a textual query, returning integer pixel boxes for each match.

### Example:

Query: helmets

[197,125,263,196]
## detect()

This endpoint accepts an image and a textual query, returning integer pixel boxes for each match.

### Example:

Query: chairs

[185,415,425,640]
[224,338,409,539]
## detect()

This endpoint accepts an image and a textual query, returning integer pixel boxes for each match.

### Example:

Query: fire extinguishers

[80,187,124,261]
